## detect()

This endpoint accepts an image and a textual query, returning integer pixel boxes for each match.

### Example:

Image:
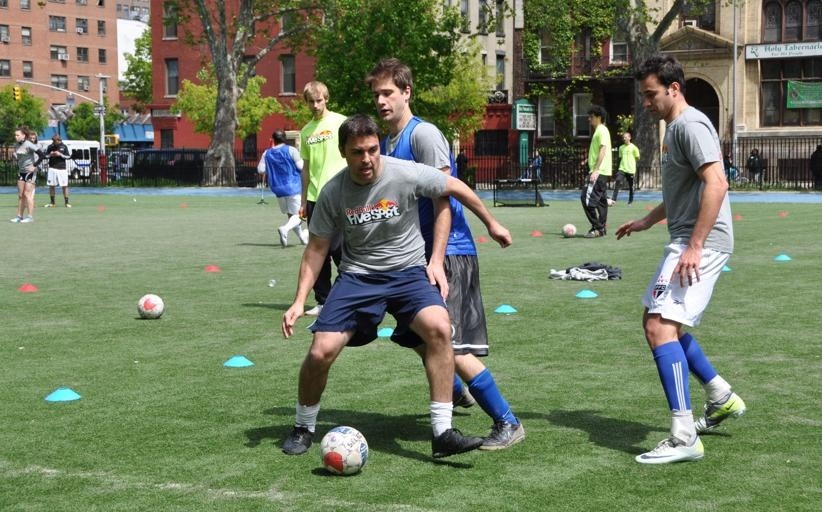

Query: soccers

[320,426,368,475]
[138,293,164,318]
[563,224,576,237]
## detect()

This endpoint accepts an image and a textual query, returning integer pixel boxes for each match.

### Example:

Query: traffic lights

[14,86,22,101]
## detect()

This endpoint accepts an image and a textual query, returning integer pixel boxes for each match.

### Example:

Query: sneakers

[583,231,599,238]
[432,386,525,458]
[694,390,746,433]
[10,216,32,223]
[283,422,313,455]
[635,434,705,464]
[277,225,289,246]
[49,203,55,207]
[65,204,71,208]
[302,228,309,246]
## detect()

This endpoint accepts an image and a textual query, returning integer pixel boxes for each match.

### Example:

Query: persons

[564,146,589,188]
[91,150,122,185]
[581,105,613,238]
[810,144,822,190]
[365,56,526,451]
[530,150,543,185]
[282,116,513,458]
[611,132,640,208]
[10,126,72,223]
[723,150,739,181]
[615,54,746,465]
[299,81,353,316]
[745,149,763,183]
[456,149,468,180]
[257,129,309,246]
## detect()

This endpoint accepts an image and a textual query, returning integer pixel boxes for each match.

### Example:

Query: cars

[101,147,259,187]
[724,166,742,184]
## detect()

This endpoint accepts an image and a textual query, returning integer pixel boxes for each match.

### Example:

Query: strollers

[517,165,535,185]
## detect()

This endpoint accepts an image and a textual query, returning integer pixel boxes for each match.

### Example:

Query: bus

[36,139,100,179]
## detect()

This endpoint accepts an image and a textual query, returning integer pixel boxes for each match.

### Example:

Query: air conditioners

[58,54,70,61]
[77,27,83,33]
[1,36,11,43]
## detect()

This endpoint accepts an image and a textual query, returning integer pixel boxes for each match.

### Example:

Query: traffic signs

[94,105,106,110]
[92,110,106,116]
[515,104,537,132]
